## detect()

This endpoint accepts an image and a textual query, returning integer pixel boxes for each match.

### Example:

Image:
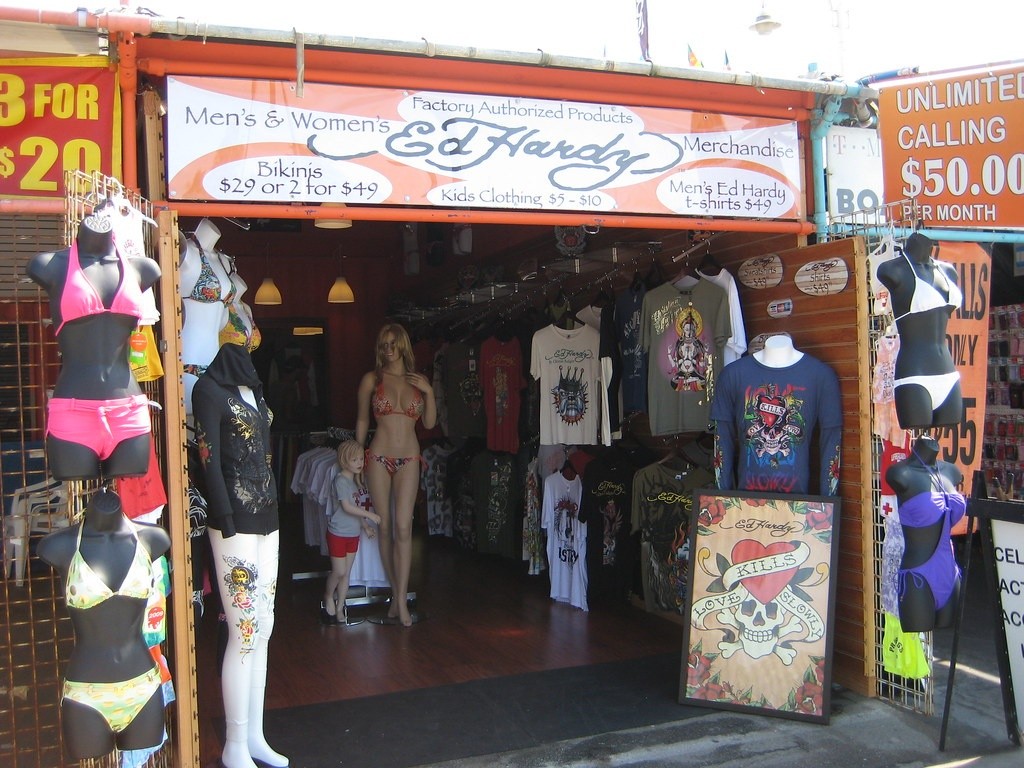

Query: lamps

[314,202,352,228]
[254,243,282,305]
[327,239,354,303]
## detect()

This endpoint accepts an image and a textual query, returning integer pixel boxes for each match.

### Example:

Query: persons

[28,219,163,484]
[193,344,291,768]
[885,435,966,631]
[36,489,170,759]
[875,233,963,426]
[353,323,437,626]
[179,215,262,613]
[324,442,381,624]
[708,332,841,497]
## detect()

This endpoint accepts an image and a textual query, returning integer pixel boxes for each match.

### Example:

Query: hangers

[409,238,733,351]
[299,425,372,471]
[441,427,716,479]
